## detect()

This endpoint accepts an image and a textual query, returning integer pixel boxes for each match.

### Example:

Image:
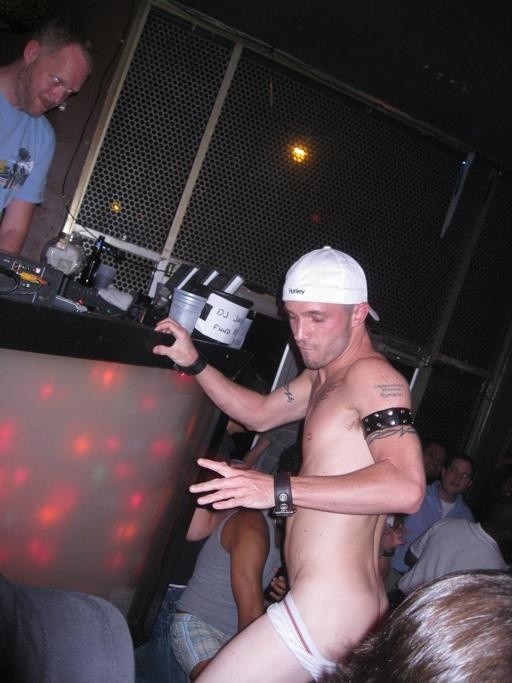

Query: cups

[167,287,207,336]
[95,263,116,289]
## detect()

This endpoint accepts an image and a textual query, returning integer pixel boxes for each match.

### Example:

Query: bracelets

[177,351,209,375]
[267,468,298,520]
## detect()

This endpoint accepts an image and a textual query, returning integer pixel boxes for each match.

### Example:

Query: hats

[281,246,380,322]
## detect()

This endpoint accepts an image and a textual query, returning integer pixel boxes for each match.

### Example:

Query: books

[211,270,245,294]
[164,263,200,292]
[195,268,221,285]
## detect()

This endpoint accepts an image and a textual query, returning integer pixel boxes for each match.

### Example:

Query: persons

[0,571,138,682]
[1,17,95,259]
[315,566,511,683]
[147,364,277,682]
[167,439,303,682]
[153,244,429,682]
[378,435,511,611]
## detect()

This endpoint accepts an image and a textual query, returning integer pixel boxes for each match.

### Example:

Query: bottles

[77,234,106,284]
